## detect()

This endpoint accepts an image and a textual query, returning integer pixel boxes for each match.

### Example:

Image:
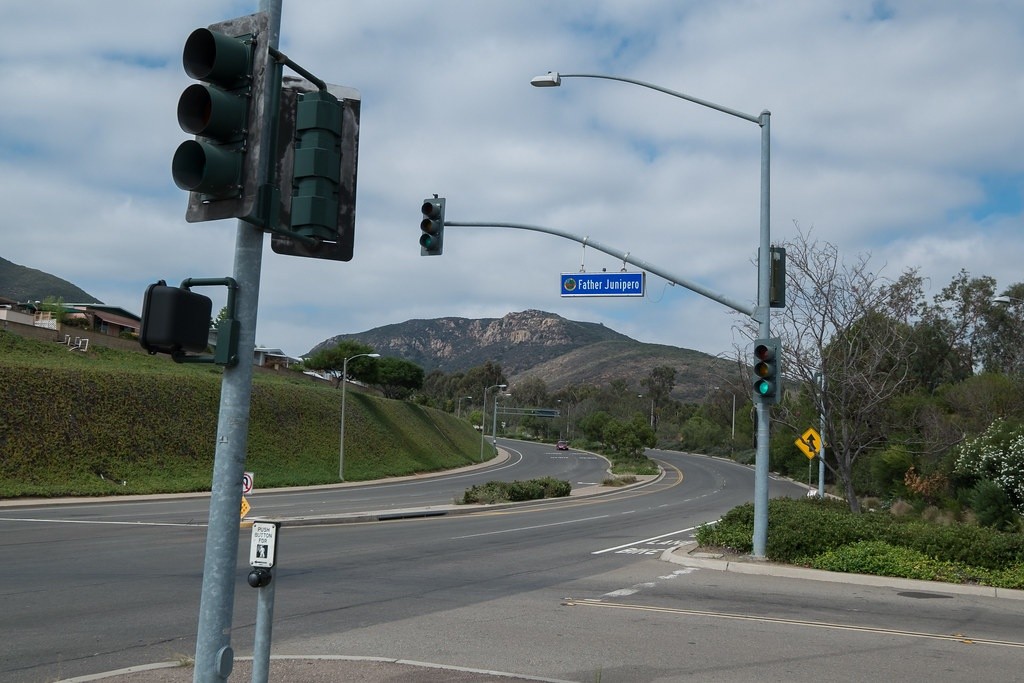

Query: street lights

[493,392,511,447]
[481,383,507,461]
[528,65,772,558]
[339,353,381,479]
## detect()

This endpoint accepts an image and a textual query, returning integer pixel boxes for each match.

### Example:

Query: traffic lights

[420,195,445,257]
[749,334,782,404]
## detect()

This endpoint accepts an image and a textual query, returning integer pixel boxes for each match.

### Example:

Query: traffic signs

[560,270,644,299]
